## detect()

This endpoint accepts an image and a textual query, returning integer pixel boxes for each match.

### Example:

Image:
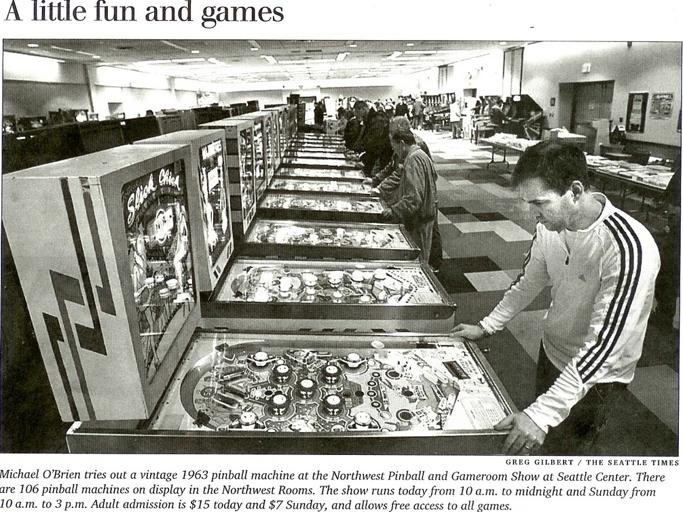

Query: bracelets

[477,322,492,338]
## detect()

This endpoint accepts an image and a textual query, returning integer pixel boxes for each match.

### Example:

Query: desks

[480,133,675,228]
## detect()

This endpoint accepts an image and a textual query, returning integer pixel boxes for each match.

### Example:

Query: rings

[524,445,532,452]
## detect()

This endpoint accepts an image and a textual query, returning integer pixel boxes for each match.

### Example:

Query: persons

[16,108,76,133]
[450,140,663,455]
[312,95,514,273]
[2,120,14,134]
